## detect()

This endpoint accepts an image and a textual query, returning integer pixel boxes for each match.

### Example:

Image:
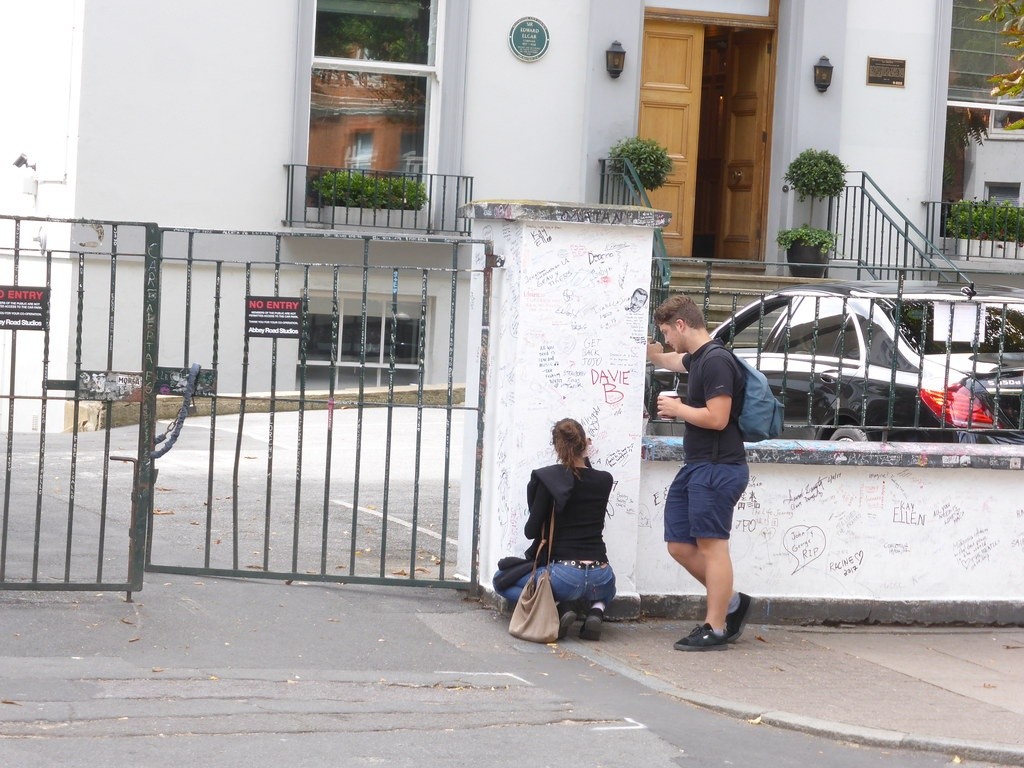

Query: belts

[557,560,608,569]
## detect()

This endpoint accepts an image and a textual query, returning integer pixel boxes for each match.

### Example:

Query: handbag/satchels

[509,574,560,643]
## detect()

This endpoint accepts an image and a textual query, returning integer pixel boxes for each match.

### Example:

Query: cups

[658,391,678,421]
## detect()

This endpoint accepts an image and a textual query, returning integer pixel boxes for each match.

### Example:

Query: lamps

[813,55,833,93]
[605,40,626,78]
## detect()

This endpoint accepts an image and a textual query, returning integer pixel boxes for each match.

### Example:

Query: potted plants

[946,196,1024,263]
[303,169,428,230]
[780,147,846,277]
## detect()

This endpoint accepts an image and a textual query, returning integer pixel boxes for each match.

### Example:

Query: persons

[645,291,751,649]
[494,417,618,641]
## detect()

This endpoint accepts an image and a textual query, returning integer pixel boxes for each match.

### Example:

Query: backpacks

[700,344,785,442]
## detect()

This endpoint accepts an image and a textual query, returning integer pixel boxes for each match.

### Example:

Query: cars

[648,285,1024,442]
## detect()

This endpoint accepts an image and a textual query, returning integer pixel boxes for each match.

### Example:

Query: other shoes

[580,608,603,640]
[558,608,577,639]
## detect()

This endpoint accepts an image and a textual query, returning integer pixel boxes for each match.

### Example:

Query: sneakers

[725,593,751,642]
[674,623,728,651]
